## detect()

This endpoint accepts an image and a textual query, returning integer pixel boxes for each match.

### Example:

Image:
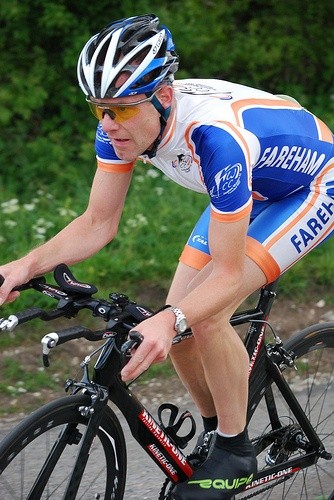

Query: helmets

[77,14,179,98]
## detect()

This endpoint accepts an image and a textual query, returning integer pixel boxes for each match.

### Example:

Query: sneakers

[171,429,256,500]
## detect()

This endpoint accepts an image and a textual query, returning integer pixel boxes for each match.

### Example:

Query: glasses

[86,83,167,125]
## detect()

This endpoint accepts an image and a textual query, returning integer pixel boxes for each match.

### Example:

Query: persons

[0,13,334,500]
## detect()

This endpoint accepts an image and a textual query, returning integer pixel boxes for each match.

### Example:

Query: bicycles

[0,262,334,499]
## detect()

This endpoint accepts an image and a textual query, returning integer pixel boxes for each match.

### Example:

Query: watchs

[166,306,187,335]
